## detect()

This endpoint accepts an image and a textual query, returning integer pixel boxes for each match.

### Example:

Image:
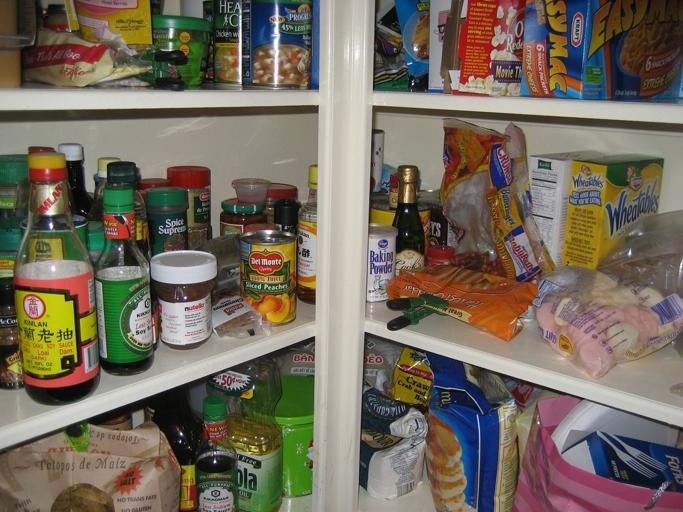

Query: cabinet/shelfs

[0,0,683,512]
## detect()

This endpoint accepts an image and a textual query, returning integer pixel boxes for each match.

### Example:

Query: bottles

[294,163,318,306]
[274,200,297,236]
[388,174,398,209]
[389,163,425,277]
[202,355,284,512]
[151,387,202,512]
[194,395,237,512]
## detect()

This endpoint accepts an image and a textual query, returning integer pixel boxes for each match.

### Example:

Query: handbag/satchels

[510,396,682,512]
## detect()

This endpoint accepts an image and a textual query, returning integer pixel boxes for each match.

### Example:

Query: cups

[230,178,270,203]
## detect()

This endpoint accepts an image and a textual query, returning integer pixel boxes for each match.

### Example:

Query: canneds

[240,229,299,325]
[369,129,385,193]
[369,199,431,267]
[219,200,268,236]
[148,249,219,350]
[424,244,453,267]
[263,182,297,224]
[203,0,312,89]
